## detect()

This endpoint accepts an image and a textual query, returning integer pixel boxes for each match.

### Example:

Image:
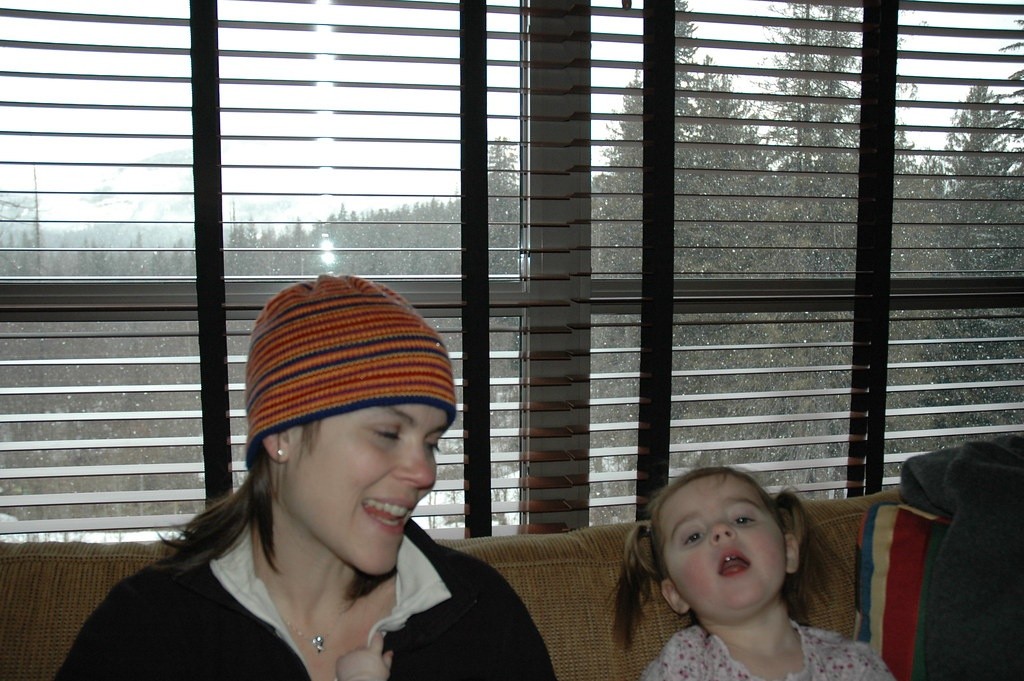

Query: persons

[613,467,896,681]
[55,275,557,681]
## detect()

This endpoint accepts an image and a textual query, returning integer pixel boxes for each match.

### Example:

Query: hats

[244,272,459,477]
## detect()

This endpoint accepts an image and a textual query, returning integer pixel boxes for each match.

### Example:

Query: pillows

[855,500,940,680]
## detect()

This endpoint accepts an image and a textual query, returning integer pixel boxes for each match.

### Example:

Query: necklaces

[282,614,338,653]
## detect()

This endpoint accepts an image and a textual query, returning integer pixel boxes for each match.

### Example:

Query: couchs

[1,487,904,681]
